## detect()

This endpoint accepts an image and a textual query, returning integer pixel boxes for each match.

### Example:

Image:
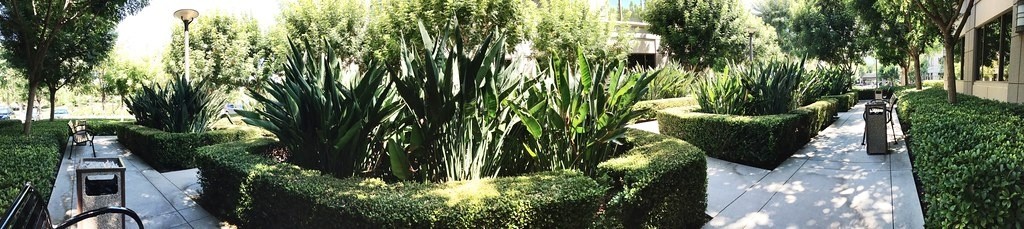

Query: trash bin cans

[75,156,127,229]
[875,88,883,100]
[865,101,888,155]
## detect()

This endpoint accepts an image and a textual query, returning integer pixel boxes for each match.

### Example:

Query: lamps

[1013,0,1024,32]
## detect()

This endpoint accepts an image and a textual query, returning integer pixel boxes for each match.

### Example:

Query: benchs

[72,120,86,135]
[0,179,145,229]
[861,92,898,145]
[67,120,97,159]
